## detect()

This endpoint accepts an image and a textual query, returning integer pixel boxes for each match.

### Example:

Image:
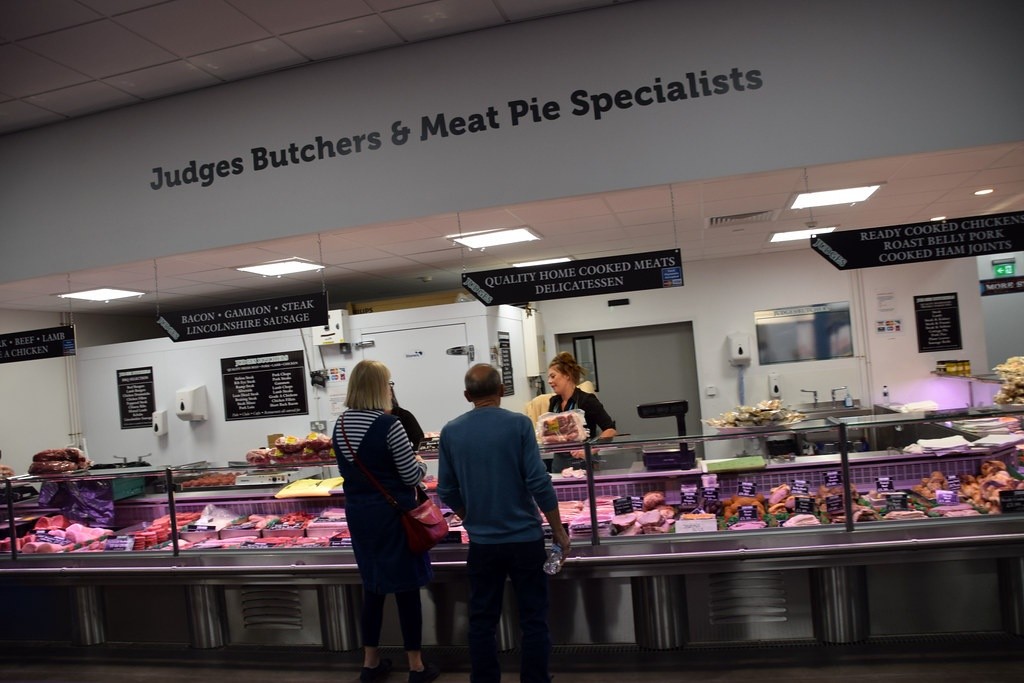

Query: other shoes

[359,658,391,682]
[409,661,440,683]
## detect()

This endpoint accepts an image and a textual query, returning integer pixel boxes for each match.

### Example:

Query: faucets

[801,388,819,409]
[831,386,847,406]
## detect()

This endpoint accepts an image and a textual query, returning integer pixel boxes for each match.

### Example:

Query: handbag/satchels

[403,499,450,551]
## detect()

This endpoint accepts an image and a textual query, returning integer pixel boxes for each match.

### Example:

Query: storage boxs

[83,474,146,503]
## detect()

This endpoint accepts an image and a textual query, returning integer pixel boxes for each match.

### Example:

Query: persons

[436,364,570,683]
[331,359,433,683]
[386,381,424,450]
[547,352,617,473]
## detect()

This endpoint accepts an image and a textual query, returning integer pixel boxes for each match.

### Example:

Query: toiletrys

[843,390,854,407]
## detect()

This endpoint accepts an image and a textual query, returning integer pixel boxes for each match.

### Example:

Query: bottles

[543,544,562,574]
[936,360,971,377]
[883,385,890,407]
